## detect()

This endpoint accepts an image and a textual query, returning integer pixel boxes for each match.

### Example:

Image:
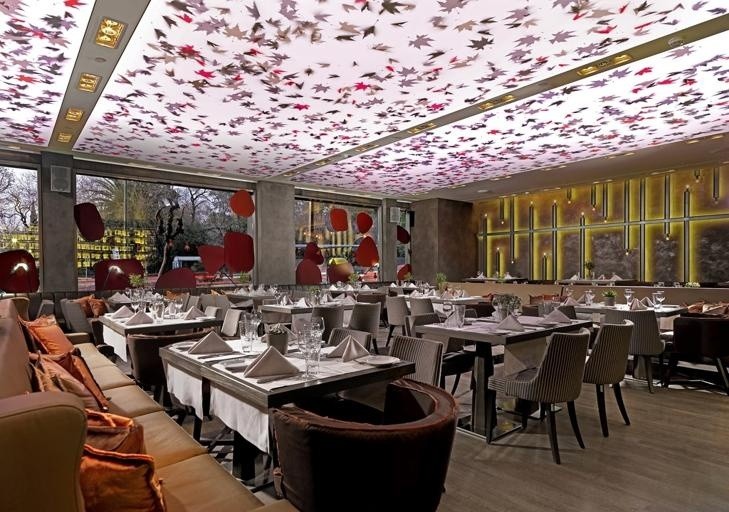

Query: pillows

[16,314,170,511]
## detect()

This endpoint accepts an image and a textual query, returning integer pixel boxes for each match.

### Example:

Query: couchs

[0,296,262,510]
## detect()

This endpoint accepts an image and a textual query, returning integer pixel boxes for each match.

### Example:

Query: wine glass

[274,289,360,309]
[446,284,462,300]
[552,287,666,311]
[251,309,263,344]
[444,297,561,331]
[248,281,279,295]
[299,331,318,379]
[126,287,156,301]
[310,317,325,335]
[131,295,183,324]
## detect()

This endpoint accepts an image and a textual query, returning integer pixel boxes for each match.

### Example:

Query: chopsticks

[198,351,238,358]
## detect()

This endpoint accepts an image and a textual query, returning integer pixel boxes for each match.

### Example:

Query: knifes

[256,371,307,384]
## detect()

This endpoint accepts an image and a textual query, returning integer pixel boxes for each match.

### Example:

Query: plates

[320,347,335,353]
[361,355,400,366]
[196,316,216,322]
[489,329,511,334]
[173,342,196,348]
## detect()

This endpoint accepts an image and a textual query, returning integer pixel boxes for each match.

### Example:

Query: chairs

[38,299,98,345]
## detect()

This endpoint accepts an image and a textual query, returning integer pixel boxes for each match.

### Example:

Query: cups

[237,320,254,351]
[658,282,664,286]
[674,282,679,287]
[304,323,320,336]
[513,281,517,284]
[610,280,615,287]
[591,272,595,280]
